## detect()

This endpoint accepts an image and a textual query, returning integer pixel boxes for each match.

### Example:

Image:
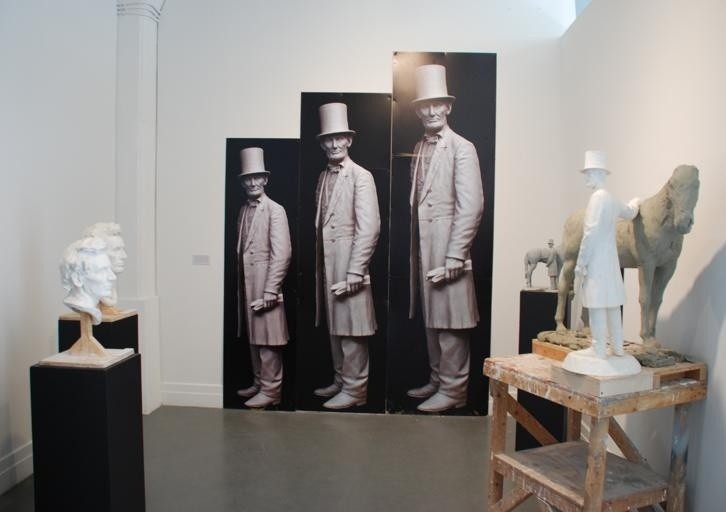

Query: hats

[409,64,456,103]
[316,102,356,138]
[582,150,610,175]
[238,147,270,177]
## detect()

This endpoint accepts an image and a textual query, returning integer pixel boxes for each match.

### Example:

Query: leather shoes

[314,384,367,409]
[238,386,281,408]
[407,384,468,412]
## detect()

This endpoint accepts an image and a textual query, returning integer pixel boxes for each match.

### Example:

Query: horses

[526,245,562,289]
[555,165,700,347]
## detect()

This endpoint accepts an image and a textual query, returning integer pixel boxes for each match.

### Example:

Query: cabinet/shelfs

[476,351,710,511]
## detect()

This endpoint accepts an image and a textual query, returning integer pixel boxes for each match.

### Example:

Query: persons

[234,148,293,407]
[83,222,127,306]
[546,239,559,289]
[61,238,116,326]
[310,103,380,409]
[573,150,641,360]
[407,64,484,412]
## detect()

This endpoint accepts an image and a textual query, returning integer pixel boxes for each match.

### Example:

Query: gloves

[250,293,284,311]
[330,275,371,296]
[427,259,473,283]
